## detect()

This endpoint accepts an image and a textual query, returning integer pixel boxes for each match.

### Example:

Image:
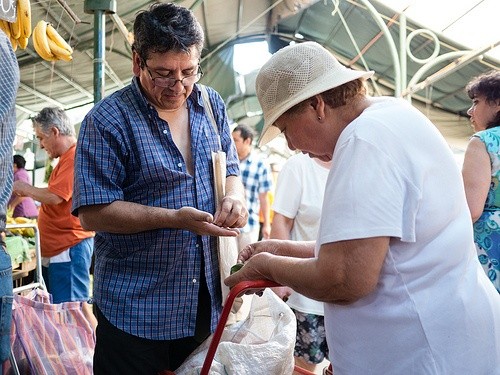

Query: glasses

[140,56,204,88]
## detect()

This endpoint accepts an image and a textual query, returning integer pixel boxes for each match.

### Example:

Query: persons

[461,69,500,295]
[7,106,98,344]
[232,124,271,253]
[0,29,21,375]
[7,155,39,286]
[270,151,332,375]
[224,41,500,374]
[71,1,250,375]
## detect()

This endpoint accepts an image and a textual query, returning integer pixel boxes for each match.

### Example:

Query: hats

[255,41,375,147]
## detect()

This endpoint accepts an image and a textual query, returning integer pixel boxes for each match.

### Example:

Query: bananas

[5,216,36,237]
[0,0,31,51]
[33,20,73,62]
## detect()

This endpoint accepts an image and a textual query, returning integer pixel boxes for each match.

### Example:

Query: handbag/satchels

[200,84,242,314]
